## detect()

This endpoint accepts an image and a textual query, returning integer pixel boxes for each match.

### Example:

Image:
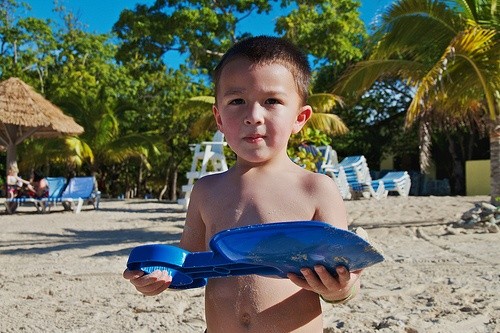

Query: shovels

[126,221,385,290]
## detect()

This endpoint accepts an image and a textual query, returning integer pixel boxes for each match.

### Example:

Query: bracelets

[319,286,356,305]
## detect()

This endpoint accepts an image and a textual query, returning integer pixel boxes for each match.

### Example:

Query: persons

[7,166,49,213]
[123,37,363,333]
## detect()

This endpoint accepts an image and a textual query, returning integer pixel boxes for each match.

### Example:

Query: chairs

[294,145,411,200]
[178,129,228,213]
[39,176,101,213]
[3,177,67,213]
